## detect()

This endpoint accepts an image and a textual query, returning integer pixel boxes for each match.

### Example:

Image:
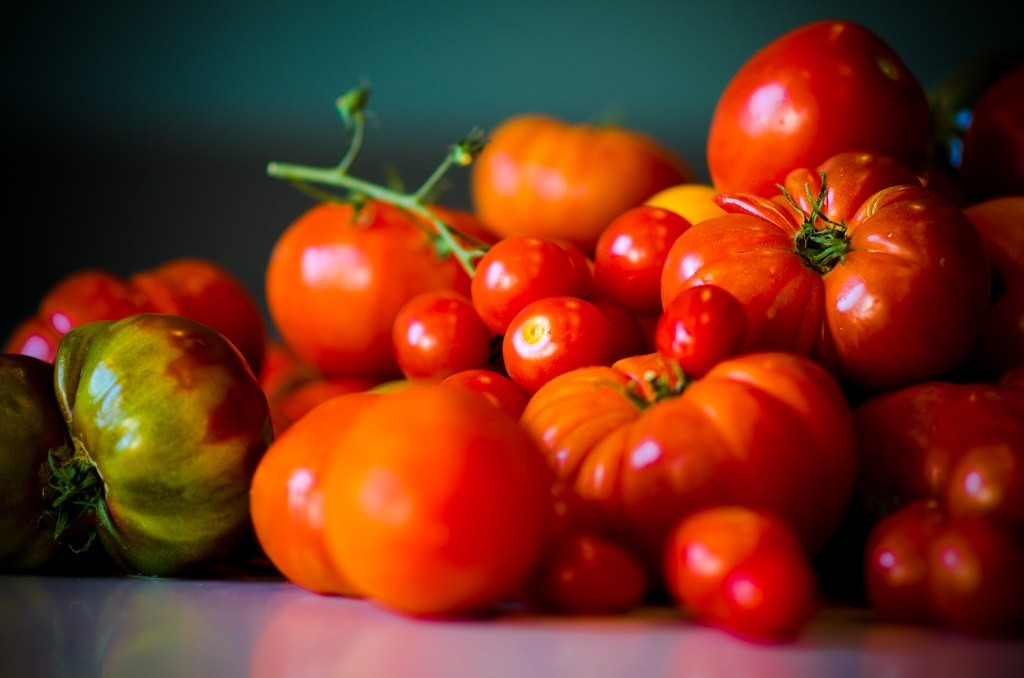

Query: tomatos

[0,15,1024,646]
